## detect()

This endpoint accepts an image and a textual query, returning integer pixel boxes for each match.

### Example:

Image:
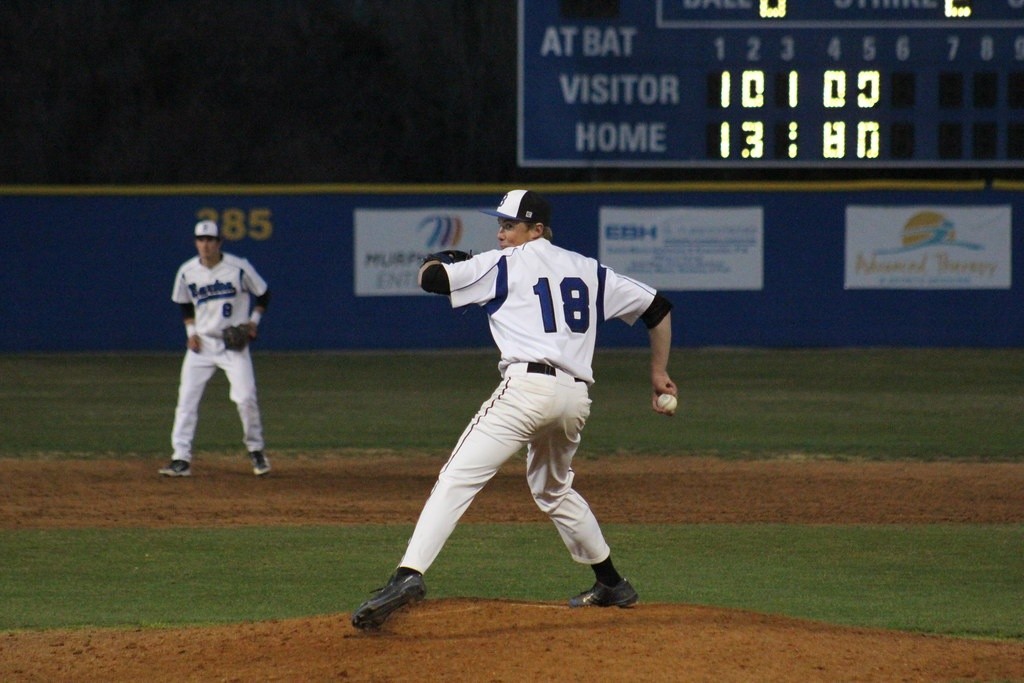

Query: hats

[194,221,220,238]
[480,188,551,227]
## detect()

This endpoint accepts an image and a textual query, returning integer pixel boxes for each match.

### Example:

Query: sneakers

[251,451,270,475]
[569,578,639,608]
[351,573,425,630]
[160,461,191,477]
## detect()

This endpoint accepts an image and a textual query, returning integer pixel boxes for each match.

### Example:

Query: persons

[350,189,679,632]
[158,219,271,476]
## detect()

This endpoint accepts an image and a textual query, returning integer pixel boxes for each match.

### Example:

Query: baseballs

[657,393,677,413]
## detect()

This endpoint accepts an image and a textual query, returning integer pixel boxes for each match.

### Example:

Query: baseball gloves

[222,323,257,353]
[419,247,475,268]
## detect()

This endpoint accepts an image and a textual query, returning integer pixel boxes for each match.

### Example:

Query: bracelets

[186,323,197,338]
[251,312,262,325]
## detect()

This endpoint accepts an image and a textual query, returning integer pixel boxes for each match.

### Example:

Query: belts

[528,362,586,382]
